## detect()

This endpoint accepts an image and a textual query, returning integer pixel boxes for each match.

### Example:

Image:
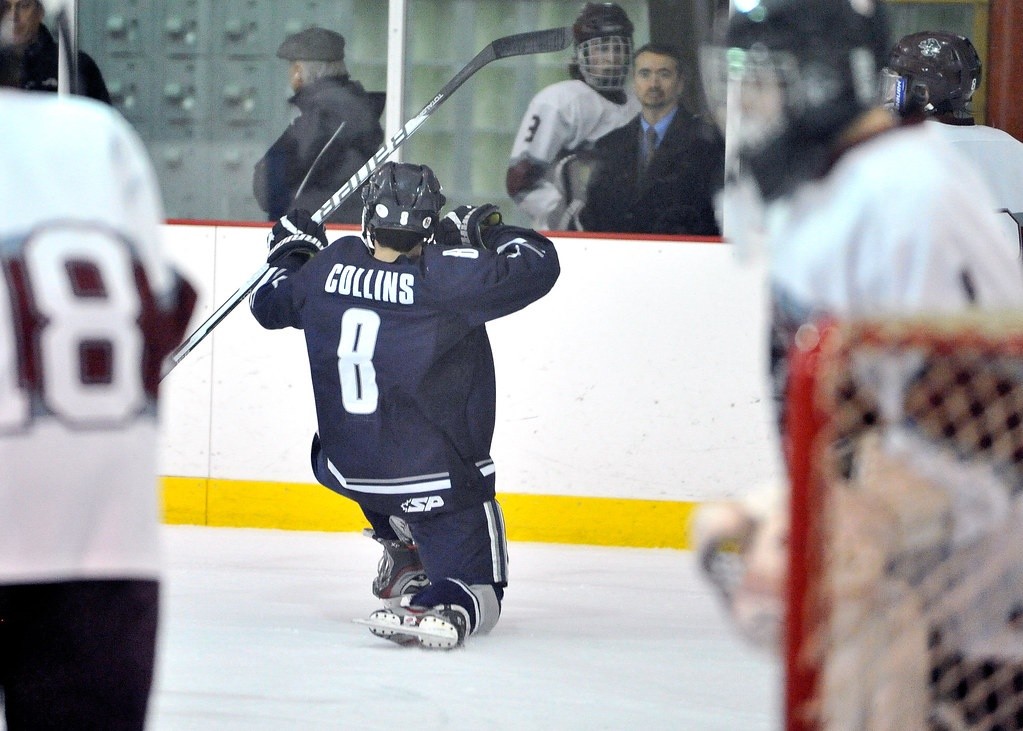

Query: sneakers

[364,528,431,609]
[351,603,468,651]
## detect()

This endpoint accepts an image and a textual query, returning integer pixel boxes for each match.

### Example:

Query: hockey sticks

[154,23,577,382]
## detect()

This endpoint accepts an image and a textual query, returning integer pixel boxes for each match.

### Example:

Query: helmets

[361,162,445,238]
[881,30,983,128]
[572,1,635,95]
[719,0,898,196]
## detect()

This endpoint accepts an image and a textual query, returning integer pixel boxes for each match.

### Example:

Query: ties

[640,127,659,176]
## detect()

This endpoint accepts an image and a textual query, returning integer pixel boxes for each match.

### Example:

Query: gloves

[545,196,587,234]
[265,207,329,273]
[428,203,504,252]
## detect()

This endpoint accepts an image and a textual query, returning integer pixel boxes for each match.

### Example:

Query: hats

[277,27,346,61]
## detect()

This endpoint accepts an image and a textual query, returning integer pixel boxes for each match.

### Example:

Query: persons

[698,0,1023,730]
[255,27,385,224]
[250,162,560,646]
[0,0,112,103]
[0,86,198,731]
[504,2,723,237]
